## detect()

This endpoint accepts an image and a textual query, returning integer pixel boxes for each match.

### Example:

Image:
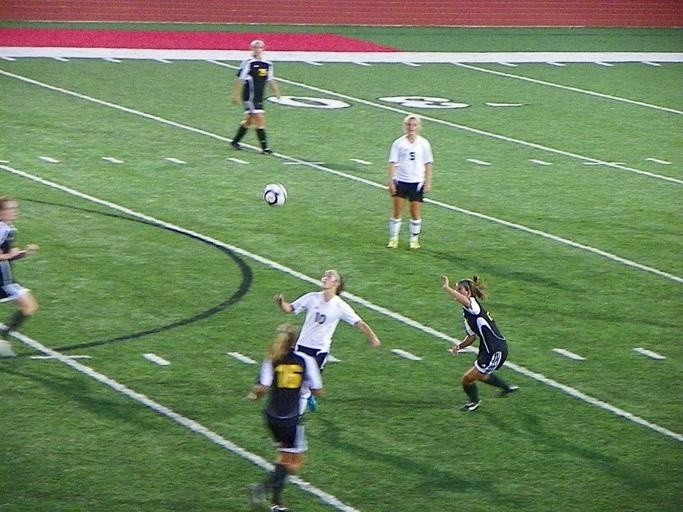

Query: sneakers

[408,240,421,249]
[387,239,399,249]
[308,395,318,412]
[460,400,481,412]
[498,385,520,395]
[0,339,17,358]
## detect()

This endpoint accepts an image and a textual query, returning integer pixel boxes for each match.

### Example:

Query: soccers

[264,183,288,208]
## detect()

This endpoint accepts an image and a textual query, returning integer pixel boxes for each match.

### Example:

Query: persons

[0,195,40,358]
[271,269,382,425]
[385,114,435,251]
[438,272,520,413]
[227,38,282,156]
[244,321,323,512]
